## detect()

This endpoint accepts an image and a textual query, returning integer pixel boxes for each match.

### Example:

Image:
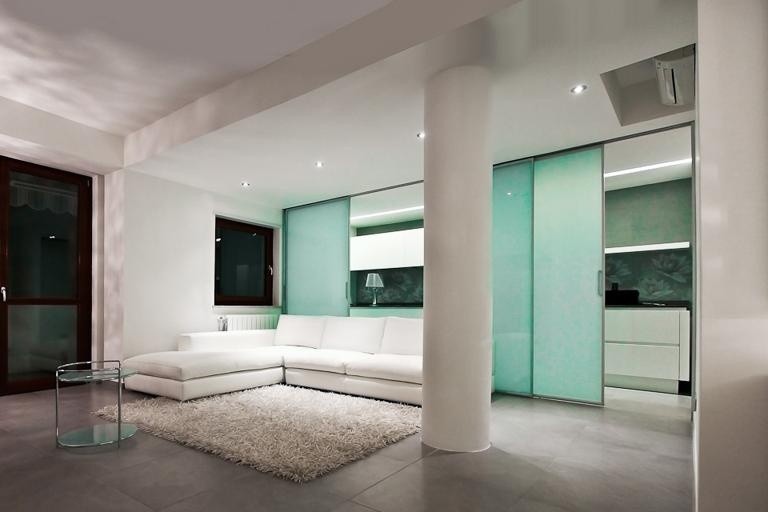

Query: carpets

[88,383,423,485]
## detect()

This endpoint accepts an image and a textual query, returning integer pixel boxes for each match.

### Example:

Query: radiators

[224,313,278,331]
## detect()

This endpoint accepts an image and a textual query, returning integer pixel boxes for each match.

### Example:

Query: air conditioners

[652,46,696,107]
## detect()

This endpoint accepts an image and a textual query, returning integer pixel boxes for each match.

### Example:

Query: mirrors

[348,181,423,316]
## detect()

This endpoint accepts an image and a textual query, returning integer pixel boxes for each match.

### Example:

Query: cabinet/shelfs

[55,359,139,451]
[604,310,680,380]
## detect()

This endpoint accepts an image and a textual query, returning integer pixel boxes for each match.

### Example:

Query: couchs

[121,313,423,406]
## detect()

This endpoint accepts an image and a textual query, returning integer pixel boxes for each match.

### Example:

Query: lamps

[365,273,384,306]
